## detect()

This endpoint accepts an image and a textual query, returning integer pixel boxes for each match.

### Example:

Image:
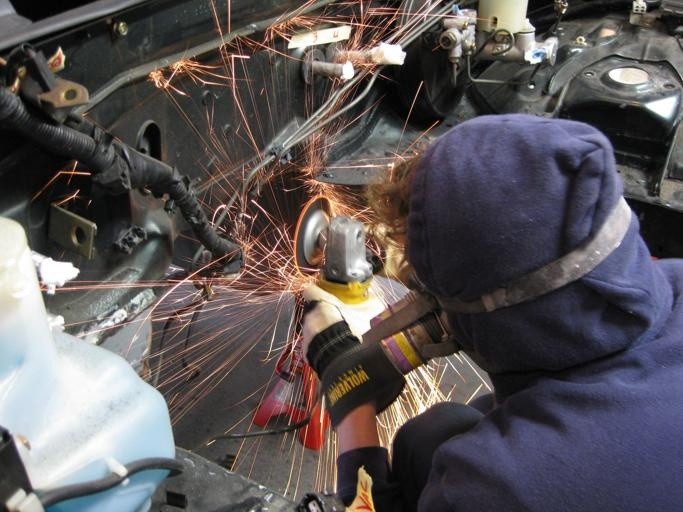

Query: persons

[296,113,681,511]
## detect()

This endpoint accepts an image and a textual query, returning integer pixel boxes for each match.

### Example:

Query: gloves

[299,283,408,428]
